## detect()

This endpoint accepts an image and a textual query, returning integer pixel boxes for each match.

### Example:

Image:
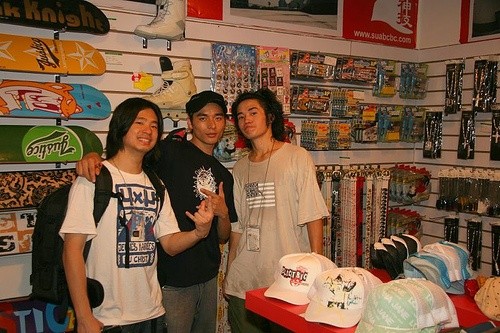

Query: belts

[315,168,391,270]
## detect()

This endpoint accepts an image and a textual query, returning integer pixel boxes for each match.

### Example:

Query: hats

[403,239,475,295]
[303,267,383,328]
[186,90,227,114]
[354,279,459,333]
[264,252,338,306]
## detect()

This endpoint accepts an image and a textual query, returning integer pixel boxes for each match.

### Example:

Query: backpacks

[29,164,165,306]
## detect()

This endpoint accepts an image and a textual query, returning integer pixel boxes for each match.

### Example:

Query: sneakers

[142,56,197,110]
[135,0,187,41]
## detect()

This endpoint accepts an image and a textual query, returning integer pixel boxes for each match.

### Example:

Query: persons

[223,87,330,333]
[57,98,214,333]
[76,90,239,333]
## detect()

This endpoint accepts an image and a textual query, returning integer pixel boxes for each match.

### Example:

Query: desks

[245,268,500,333]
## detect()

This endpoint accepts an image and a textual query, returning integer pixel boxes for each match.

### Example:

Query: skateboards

[0,0,110,36]
[0,79,111,120]
[0,125,103,164]
[0,168,79,208]
[0,33,106,76]
[0,296,93,333]
[0,209,41,257]
